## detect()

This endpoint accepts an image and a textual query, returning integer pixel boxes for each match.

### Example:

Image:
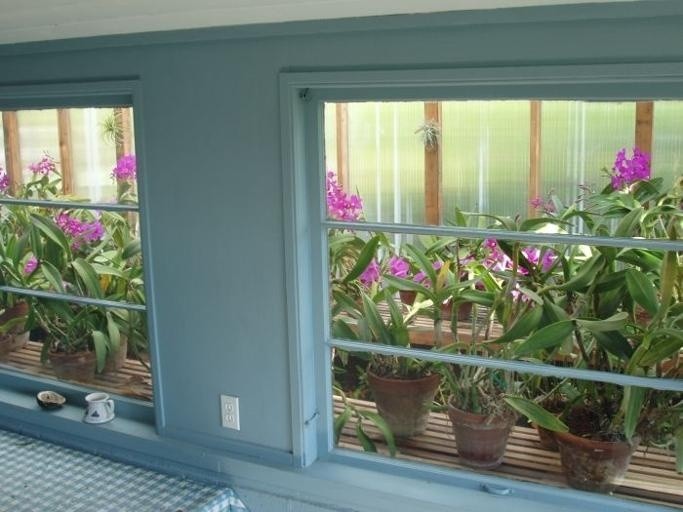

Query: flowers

[0,154,152,385]
[316,147,683,476]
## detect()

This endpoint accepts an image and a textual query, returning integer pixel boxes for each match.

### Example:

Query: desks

[1,429,234,511]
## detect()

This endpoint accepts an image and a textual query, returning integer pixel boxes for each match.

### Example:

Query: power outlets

[219,394,241,431]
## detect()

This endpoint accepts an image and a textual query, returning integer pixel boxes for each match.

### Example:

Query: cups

[83,392,116,425]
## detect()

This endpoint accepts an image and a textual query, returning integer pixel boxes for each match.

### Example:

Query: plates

[37,390,65,410]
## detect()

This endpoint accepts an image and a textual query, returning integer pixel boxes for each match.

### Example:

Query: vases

[552,405,643,495]
